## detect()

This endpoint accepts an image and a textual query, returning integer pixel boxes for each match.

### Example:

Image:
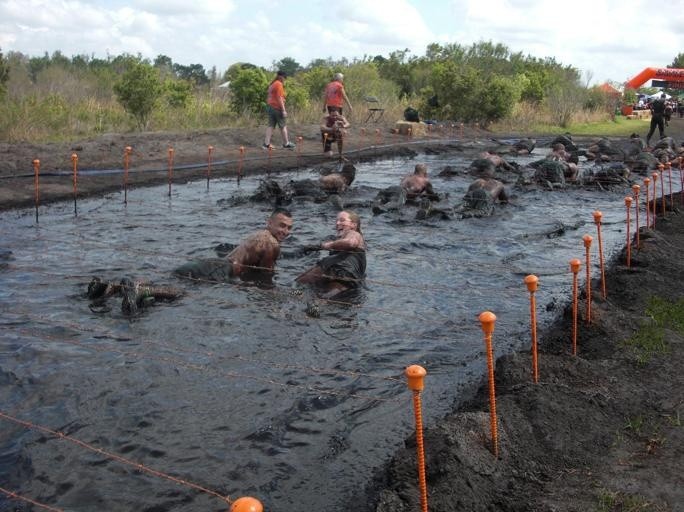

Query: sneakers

[262,143,275,149]
[281,142,296,149]
[119,278,138,313]
[88,277,110,300]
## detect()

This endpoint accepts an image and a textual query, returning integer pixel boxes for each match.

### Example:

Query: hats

[278,70,288,79]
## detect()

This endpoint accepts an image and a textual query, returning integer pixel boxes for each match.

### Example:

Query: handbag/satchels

[405,108,419,123]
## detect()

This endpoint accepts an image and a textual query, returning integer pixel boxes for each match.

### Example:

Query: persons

[261,68,296,151]
[633,94,684,126]
[644,94,667,141]
[320,105,352,162]
[89,207,308,315]
[418,170,510,221]
[253,162,356,203]
[322,71,352,115]
[295,208,367,316]
[437,130,683,192]
[343,164,442,217]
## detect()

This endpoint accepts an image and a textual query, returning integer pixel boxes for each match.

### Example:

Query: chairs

[363,94,386,124]
